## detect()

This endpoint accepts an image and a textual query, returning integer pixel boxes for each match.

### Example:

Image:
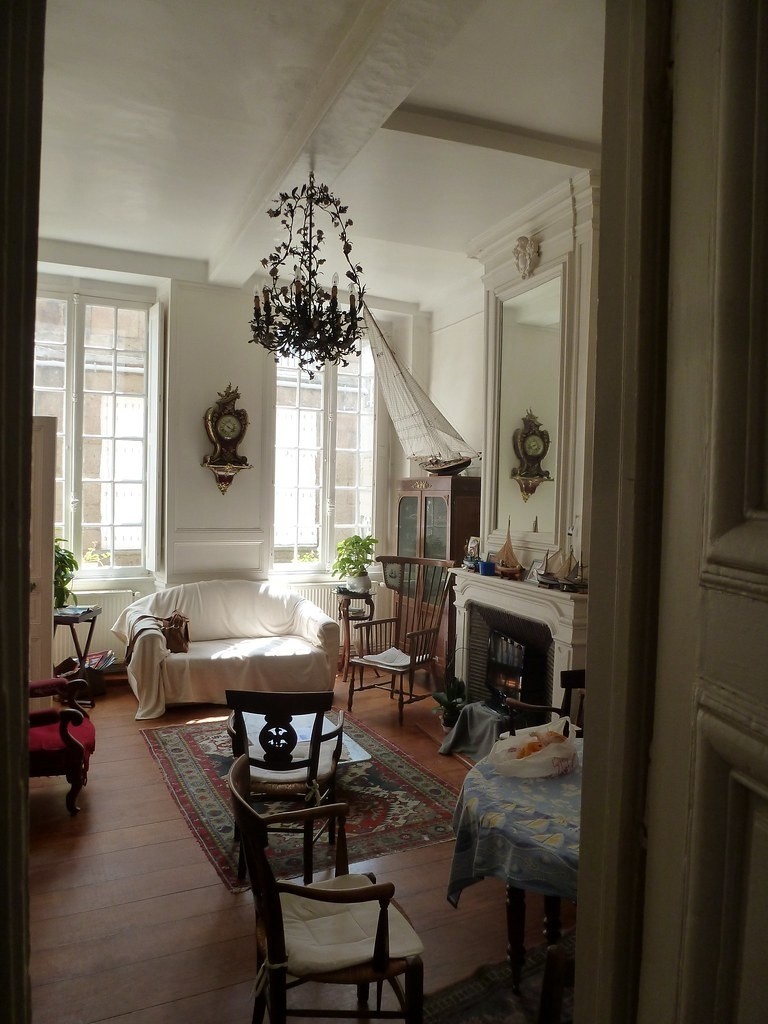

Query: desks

[446,735,584,991]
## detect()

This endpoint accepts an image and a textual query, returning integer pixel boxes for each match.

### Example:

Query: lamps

[249,173,366,379]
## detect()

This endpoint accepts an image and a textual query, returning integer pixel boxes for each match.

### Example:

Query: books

[74,650,118,673]
[60,604,100,615]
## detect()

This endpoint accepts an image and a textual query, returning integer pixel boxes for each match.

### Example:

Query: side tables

[338,591,381,682]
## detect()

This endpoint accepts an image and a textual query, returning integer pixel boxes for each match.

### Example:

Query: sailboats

[534,544,580,592]
[362,300,482,474]
[492,513,525,576]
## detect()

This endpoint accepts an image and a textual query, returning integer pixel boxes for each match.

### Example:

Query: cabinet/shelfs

[390,473,481,690]
[52,602,102,692]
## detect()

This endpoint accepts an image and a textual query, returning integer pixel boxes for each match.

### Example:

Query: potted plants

[53,538,77,608]
[331,534,379,594]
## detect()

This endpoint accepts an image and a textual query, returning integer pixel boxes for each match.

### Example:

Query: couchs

[111,579,341,721]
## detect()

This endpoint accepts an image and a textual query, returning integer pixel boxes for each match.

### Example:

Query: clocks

[510,409,554,503]
[199,382,255,496]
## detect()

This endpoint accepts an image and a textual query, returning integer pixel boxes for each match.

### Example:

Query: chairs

[504,668,587,738]
[225,688,349,888]
[347,555,460,725]
[28,677,95,817]
[228,752,426,1024]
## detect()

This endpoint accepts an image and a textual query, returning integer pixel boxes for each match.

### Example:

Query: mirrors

[479,236,573,559]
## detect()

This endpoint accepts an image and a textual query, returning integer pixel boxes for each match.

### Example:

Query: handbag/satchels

[123,609,191,666]
[483,716,578,778]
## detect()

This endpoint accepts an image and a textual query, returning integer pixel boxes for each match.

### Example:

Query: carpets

[424,922,574,1024]
[138,705,460,895]
